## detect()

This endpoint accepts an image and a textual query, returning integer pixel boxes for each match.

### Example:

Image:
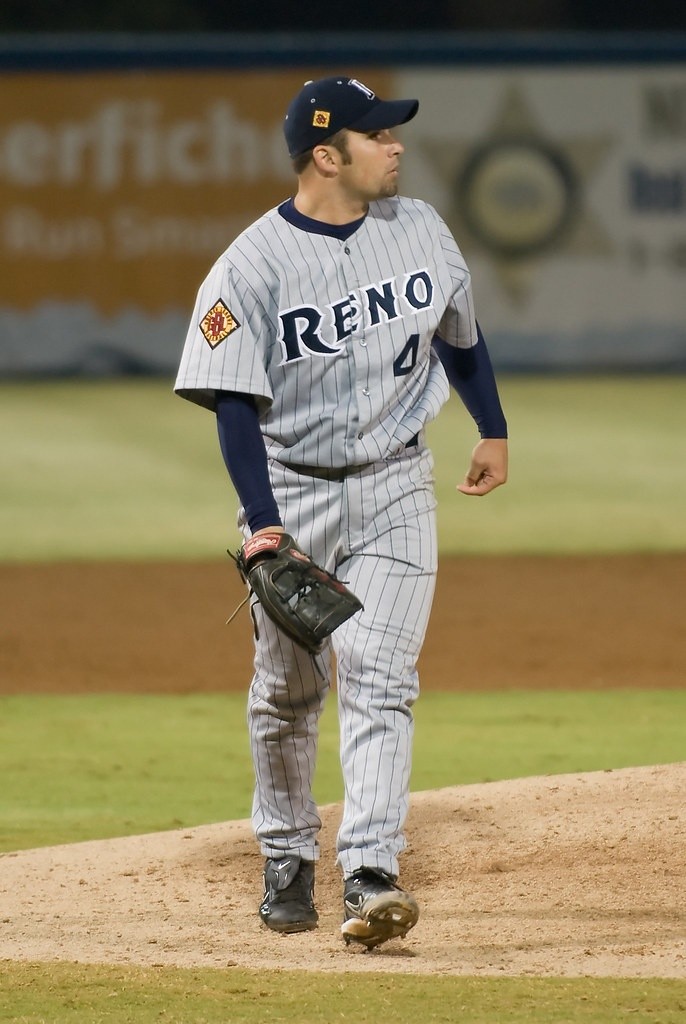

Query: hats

[284,76,420,158]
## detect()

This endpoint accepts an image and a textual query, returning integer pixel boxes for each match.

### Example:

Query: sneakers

[342,868,421,951]
[259,855,319,936]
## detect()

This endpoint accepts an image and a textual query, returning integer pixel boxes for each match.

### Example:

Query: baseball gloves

[236,532,365,654]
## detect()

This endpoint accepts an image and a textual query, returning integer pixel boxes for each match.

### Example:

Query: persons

[176,76,508,950]
